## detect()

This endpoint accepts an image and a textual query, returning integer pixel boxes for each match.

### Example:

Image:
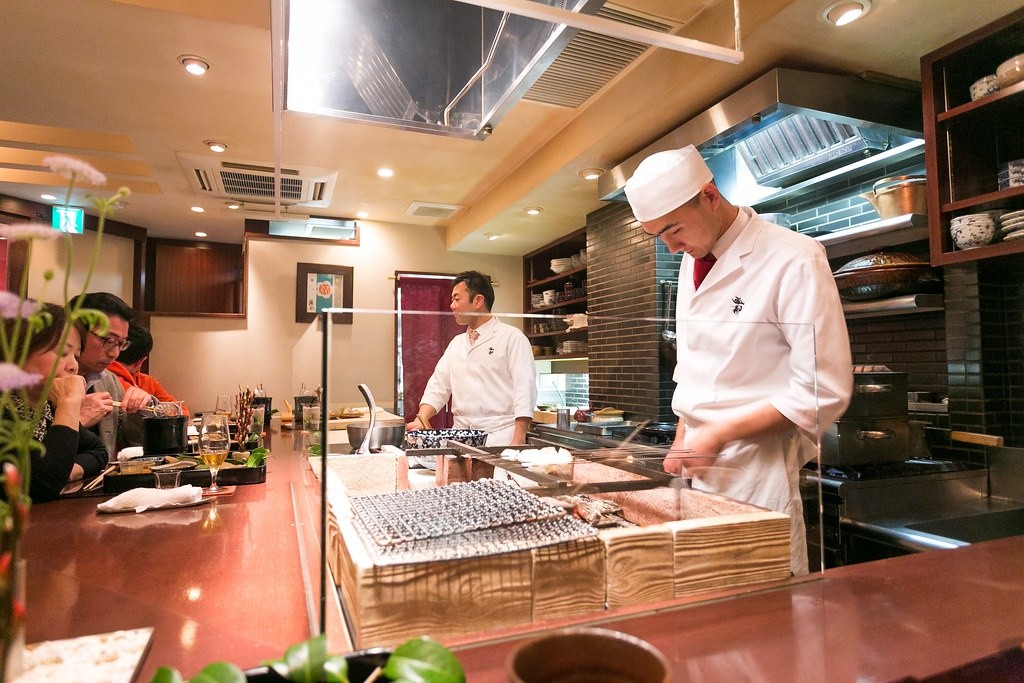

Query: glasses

[87,328,131,352]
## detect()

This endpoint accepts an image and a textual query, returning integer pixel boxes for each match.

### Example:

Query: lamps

[177,53,211,76]
[224,200,243,211]
[819,0,871,28]
[203,139,228,152]
[579,168,605,180]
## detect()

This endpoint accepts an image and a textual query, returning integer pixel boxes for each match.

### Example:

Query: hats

[622,143,713,221]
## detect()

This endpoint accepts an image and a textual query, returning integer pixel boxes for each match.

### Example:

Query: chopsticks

[114,401,154,412]
[83,464,116,490]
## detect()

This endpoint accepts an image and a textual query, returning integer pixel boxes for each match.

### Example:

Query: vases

[0,501,31,682]
[232,452,250,459]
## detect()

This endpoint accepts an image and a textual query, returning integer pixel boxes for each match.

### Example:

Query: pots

[346,422,406,448]
[141,416,188,455]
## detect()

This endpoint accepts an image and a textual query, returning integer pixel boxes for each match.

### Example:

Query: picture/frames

[295,262,353,324]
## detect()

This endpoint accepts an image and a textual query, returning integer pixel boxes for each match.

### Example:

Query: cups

[557,408,570,426]
[543,291,555,306]
[153,470,181,489]
[119,462,155,473]
[215,395,232,424]
[303,406,320,430]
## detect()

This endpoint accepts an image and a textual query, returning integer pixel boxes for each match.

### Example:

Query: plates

[999,210,1024,241]
[550,258,573,273]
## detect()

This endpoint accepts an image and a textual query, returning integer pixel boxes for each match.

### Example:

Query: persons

[0,293,190,504]
[623,144,853,576]
[404,272,537,447]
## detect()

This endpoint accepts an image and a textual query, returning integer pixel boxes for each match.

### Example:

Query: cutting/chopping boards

[328,409,404,429]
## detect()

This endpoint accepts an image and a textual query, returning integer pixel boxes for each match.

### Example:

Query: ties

[469,330,479,347]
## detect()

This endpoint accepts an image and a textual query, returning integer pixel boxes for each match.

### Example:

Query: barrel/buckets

[860,173,927,219]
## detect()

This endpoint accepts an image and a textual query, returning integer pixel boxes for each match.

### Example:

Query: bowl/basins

[833,253,930,301]
[151,462,197,471]
[594,408,624,423]
[507,626,668,683]
[969,74,997,100]
[543,347,553,355]
[537,406,549,411]
[574,410,593,422]
[996,53,1024,86]
[132,457,164,466]
[950,209,1013,250]
[159,401,180,416]
[532,346,542,356]
[407,428,487,471]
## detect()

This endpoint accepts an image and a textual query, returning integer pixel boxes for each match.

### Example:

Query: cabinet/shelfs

[920,5,1024,268]
[523,226,588,360]
[750,132,944,320]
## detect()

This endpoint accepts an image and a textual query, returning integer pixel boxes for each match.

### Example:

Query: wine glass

[199,414,231,493]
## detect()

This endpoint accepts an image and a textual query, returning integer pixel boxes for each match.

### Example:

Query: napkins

[95,483,201,513]
[117,445,144,462]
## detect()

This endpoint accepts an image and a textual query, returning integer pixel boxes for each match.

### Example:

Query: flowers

[233,383,257,452]
[0,157,129,515]
